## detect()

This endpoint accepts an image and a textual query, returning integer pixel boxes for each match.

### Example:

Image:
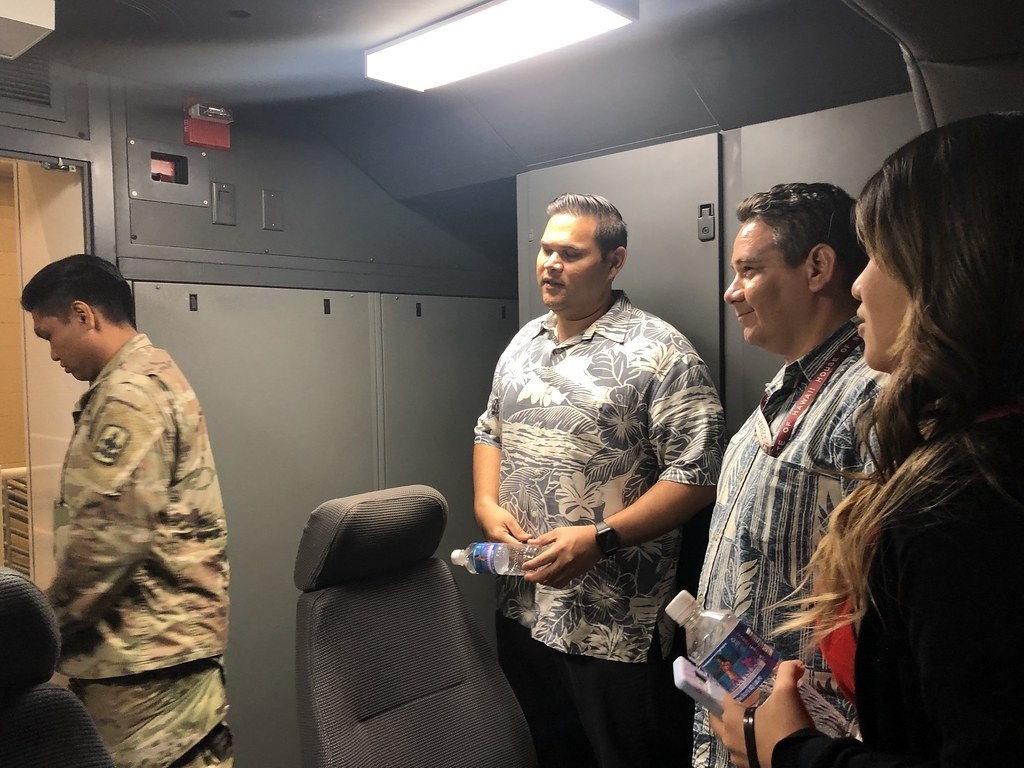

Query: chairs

[0,568,118,768]
[293,484,542,767]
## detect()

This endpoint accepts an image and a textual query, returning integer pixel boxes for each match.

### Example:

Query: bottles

[451,542,551,576]
[664,589,863,743]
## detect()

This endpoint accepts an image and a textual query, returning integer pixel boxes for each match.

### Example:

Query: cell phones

[673,656,729,719]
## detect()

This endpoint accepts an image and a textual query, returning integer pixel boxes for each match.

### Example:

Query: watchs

[592,520,622,557]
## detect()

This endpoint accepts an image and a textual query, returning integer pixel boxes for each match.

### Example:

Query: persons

[690,178,895,768]
[20,254,238,768]
[709,113,1024,768]
[471,194,723,768]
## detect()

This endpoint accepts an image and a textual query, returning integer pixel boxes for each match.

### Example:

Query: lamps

[0,0,56,61]
[364,0,640,93]
